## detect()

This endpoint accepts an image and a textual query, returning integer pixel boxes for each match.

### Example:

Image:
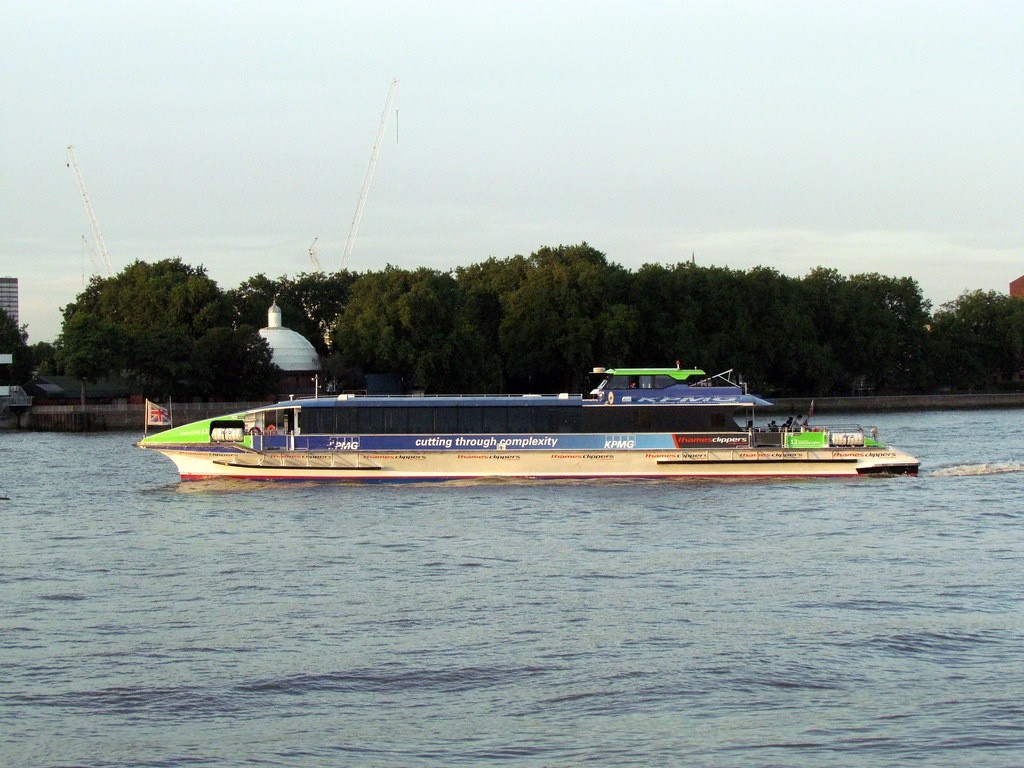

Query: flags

[147,401,172,426]
[808,400,816,416]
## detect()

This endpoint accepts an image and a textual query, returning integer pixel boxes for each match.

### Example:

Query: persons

[782,417,793,428]
[788,414,802,432]
[768,421,779,432]
[630,381,636,388]
[747,420,753,430]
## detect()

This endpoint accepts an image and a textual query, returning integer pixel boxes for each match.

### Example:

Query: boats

[134,359,922,487]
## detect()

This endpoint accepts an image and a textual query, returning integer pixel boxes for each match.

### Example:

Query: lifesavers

[266,425,277,435]
[249,427,261,435]
